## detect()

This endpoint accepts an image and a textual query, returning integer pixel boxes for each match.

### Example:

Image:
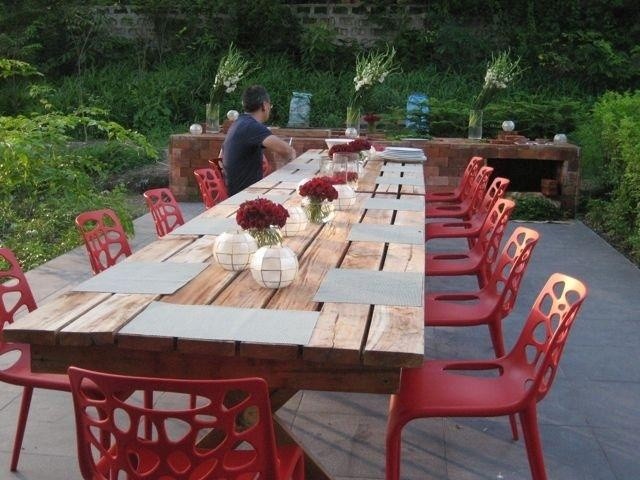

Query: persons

[216,84,296,199]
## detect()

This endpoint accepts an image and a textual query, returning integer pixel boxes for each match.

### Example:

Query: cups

[319,152,359,182]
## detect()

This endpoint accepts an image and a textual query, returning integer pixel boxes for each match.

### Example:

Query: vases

[204,104,219,132]
[468,110,483,139]
[346,108,361,137]
[368,121,375,131]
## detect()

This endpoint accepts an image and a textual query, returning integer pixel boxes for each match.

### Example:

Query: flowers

[205,40,256,115]
[347,41,403,126]
[364,112,380,121]
[468,46,532,125]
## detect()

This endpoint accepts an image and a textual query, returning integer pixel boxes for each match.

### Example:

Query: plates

[383,147,427,163]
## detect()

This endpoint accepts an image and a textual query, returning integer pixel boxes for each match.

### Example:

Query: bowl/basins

[324,138,354,152]
